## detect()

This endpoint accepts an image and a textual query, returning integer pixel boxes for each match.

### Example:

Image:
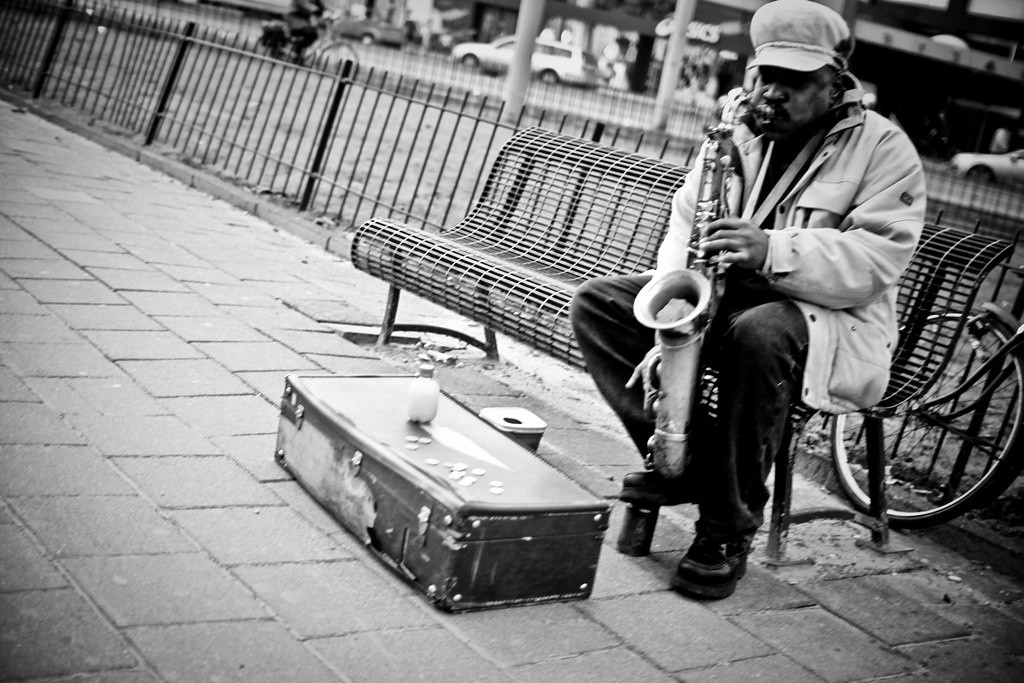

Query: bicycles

[252,10,359,84]
[828,260,1023,529]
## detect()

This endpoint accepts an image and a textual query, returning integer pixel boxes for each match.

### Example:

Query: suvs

[451,35,601,92]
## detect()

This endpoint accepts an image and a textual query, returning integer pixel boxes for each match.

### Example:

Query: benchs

[348,123,1014,565]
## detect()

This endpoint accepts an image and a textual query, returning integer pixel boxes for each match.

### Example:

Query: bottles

[408,363,440,424]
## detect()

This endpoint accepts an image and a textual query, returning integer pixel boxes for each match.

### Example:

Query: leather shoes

[620,463,703,509]
[672,526,754,600]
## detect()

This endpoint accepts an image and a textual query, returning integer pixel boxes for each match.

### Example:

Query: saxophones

[630,85,771,481]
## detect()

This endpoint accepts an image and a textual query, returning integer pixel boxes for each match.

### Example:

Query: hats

[746,0,851,72]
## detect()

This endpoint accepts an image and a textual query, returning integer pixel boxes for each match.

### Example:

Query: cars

[949,146,1023,188]
[334,14,405,49]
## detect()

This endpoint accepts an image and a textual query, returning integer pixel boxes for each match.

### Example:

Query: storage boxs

[273,373,615,614]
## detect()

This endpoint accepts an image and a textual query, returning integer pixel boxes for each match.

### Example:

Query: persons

[571,0,926,598]
[284,0,337,67]
